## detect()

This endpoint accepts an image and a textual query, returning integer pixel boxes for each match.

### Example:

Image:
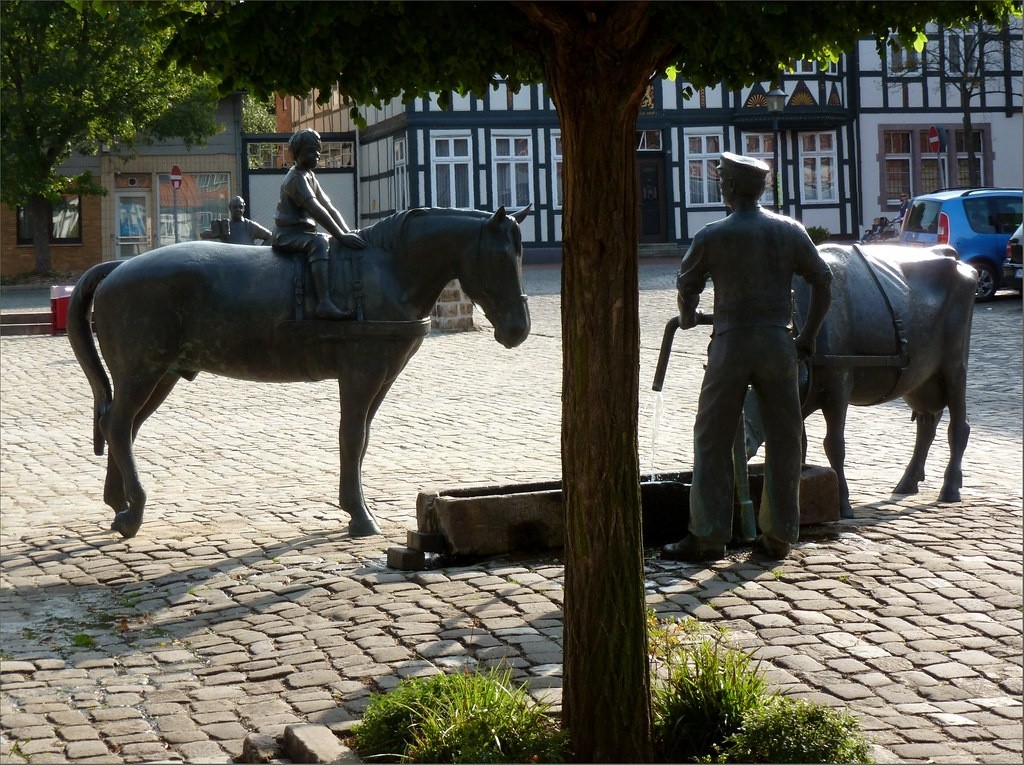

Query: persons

[203,194,275,247]
[895,191,910,229]
[272,128,369,317]
[661,151,834,563]
[860,217,882,241]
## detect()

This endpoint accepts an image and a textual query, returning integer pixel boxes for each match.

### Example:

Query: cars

[1003,223,1023,297]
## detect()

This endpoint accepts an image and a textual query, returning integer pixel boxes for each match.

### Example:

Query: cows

[736,239,978,518]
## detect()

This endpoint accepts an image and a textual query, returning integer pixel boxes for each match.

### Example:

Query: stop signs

[928,125,941,154]
[170,166,182,189]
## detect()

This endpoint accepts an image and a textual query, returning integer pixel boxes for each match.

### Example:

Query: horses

[64,203,532,539]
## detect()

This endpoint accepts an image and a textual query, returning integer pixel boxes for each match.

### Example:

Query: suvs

[899,186,1024,303]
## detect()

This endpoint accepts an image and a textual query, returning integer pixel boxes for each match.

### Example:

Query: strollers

[862,216,902,246]
